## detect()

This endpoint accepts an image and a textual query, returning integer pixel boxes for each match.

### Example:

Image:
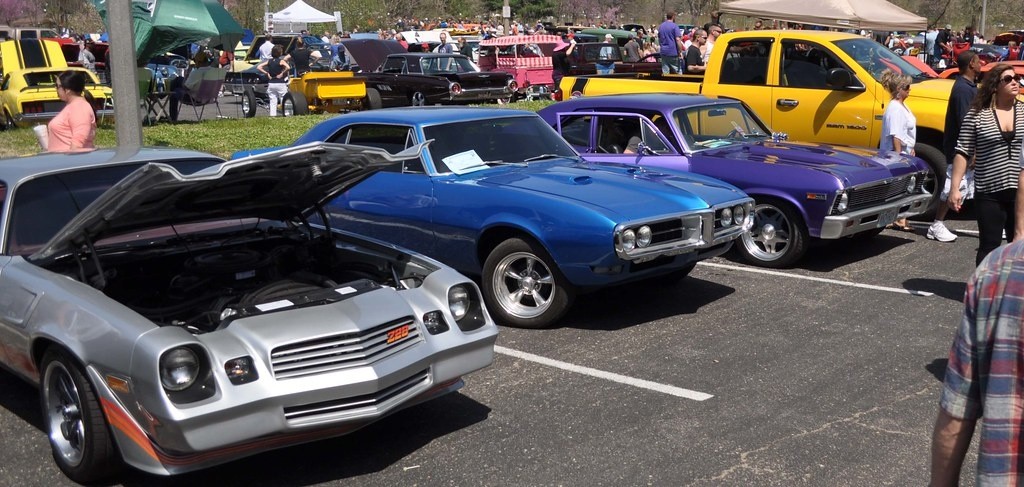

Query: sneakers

[927,224,957,242]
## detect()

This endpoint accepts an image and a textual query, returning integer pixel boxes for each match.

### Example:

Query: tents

[273,0,338,36]
[716,0,927,64]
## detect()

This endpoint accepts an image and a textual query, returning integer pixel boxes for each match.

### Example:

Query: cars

[225,34,295,95]
[241,67,382,117]
[0,139,500,486]
[400,29,480,69]
[0,37,115,126]
[146,56,188,93]
[230,106,756,326]
[339,38,519,108]
[502,93,935,270]
[224,49,331,98]
[39,38,110,88]
[451,25,708,95]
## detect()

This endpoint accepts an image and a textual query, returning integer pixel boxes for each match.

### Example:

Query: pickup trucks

[556,29,1023,224]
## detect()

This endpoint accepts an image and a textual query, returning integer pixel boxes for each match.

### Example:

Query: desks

[147,91,175,125]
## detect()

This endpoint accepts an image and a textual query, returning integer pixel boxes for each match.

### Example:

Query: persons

[1012,139,1024,244]
[355,10,805,91]
[948,64,1024,268]
[46,71,96,152]
[879,25,1024,243]
[928,241,1023,487]
[169,30,349,124]
[805,24,881,42]
[79,41,94,74]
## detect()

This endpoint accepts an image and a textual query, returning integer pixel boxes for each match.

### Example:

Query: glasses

[900,85,909,90]
[55,83,65,90]
[997,75,1022,83]
[701,37,707,39]
[714,30,721,33]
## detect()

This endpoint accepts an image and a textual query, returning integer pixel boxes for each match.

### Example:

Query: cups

[34,124,49,150]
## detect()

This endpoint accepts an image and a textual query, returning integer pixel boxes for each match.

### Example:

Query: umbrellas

[93,0,247,121]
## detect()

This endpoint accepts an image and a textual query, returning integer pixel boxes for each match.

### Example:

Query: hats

[191,51,207,61]
[605,33,614,38]
[554,40,571,51]
[650,24,656,27]
[945,24,951,29]
[338,45,345,53]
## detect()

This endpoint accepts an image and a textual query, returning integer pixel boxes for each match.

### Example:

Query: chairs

[783,48,818,89]
[600,121,636,152]
[101,67,228,126]
[652,116,673,149]
